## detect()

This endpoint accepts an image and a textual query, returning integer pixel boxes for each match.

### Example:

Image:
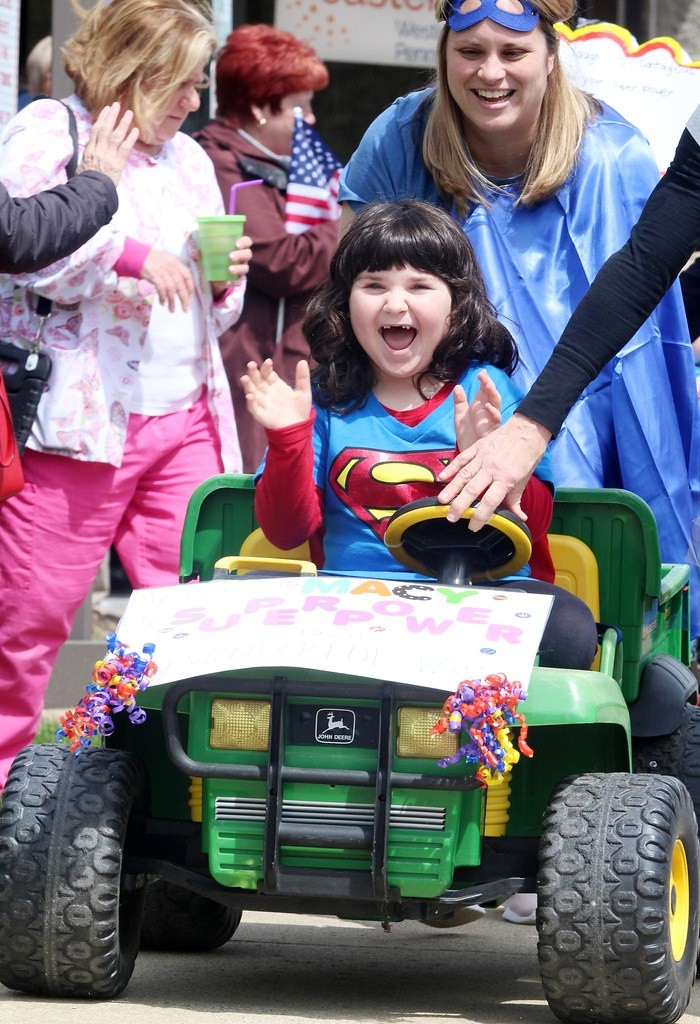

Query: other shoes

[504,893,536,924]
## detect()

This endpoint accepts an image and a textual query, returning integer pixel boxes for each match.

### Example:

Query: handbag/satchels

[1,365,23,501]
[1,336,54,456]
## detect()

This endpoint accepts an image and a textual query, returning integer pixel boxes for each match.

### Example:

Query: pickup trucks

[0,472,700,1024]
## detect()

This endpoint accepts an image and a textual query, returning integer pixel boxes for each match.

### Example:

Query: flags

[283,107,345,234]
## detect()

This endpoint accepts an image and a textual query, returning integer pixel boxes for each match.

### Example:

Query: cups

[196,214,246,281]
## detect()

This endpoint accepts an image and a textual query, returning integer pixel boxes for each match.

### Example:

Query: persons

[436,109,700,533]
[0,0,700,923]
[240,194,598,673]
